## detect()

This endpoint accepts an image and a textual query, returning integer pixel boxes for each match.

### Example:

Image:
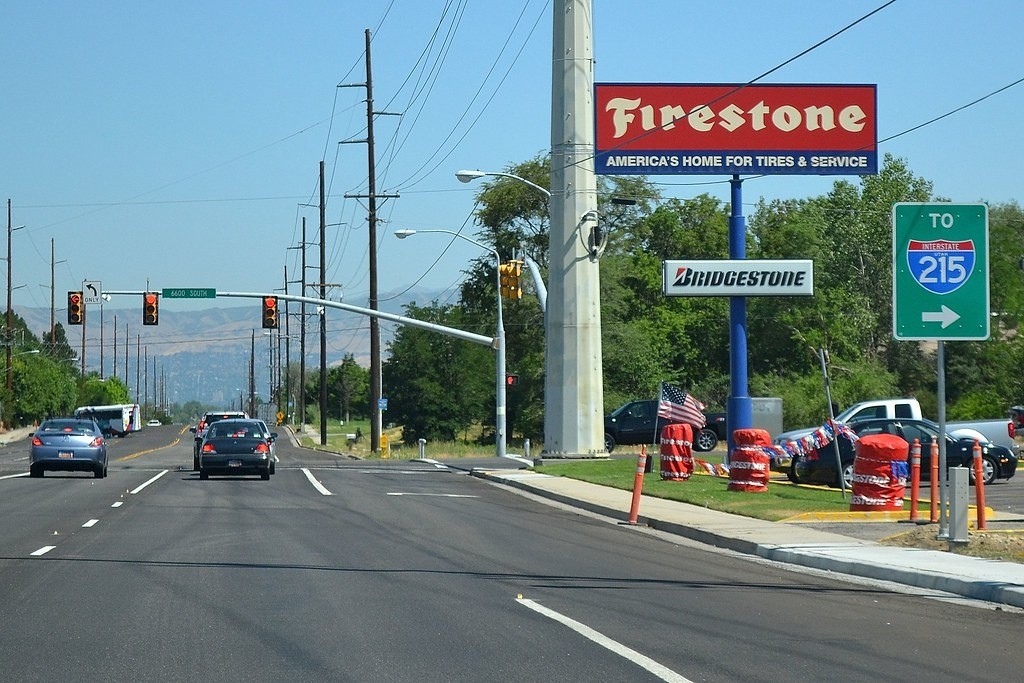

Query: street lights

[236,388,255,419]
[394,228,506,456]
[254,392,276,403]
[6,350,40,430]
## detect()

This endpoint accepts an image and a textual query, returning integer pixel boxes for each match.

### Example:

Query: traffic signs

[82,281,101,305]
[891,202,990,342]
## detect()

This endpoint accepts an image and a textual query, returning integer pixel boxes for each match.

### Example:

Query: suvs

[189,412,251,469]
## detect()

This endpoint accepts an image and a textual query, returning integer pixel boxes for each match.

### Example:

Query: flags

[657,383,708,435]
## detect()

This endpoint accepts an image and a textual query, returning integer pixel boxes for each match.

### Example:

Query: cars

[28,418,110,478]
[798,416,1018,488]
[213,419,280,474]
[146,420,163,426]
[195,419,275,480]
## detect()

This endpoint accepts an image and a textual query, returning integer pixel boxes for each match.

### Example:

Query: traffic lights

[143,293,158,325]
[68,292,84,325]
[262,297,278,329]
[507,264,521,301]
[506,375,520,386]
[499,263,510,296]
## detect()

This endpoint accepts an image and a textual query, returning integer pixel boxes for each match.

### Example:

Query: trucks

[256,403,278,426]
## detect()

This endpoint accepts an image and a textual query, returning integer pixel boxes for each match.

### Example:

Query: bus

[73,403,142,438]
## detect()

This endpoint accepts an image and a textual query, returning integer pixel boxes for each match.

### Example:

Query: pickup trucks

[768,398,1020,482]
[604,400,728,452]
